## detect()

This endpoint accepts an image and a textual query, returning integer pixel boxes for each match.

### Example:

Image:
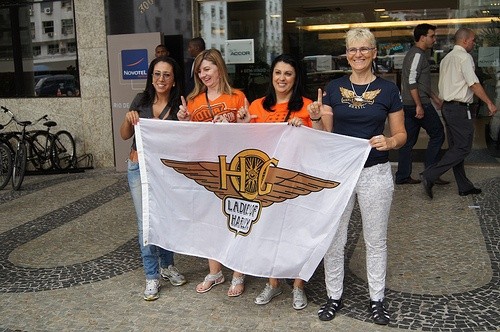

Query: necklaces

[349,72,373,99]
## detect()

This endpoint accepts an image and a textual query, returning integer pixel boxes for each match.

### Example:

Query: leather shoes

[395,175,421,184]
[423,171,433,200]
[435,178,450,184]
[459,188,481,196]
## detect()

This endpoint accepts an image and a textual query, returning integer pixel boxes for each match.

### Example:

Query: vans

[33,74,77,97]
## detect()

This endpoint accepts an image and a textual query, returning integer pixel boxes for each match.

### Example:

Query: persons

[307,28,408,326]
[394,22,453,188]
[120,55,189,304]
[181,36,207,101]
[153,42,172,59]
[419,26,498,200]
[234,53,318,311]
[176,48,252,298]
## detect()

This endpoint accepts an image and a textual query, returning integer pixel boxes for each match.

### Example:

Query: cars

[302,50,445,97]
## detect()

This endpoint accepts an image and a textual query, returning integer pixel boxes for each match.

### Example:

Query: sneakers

[254,283,282,305]
[161,264,186,286]
[144,278,161,301]
[292,287,307,310]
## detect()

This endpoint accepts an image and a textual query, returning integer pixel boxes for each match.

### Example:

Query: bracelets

[310,116,321,122]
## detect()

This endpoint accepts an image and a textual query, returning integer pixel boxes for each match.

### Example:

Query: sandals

[227,275,245,297]
[318,298,344,321]
[370,299,390,324]
[196,270,224,293]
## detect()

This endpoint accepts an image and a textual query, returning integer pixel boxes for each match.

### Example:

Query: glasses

[151,72,174,80]
[346,47,377,53]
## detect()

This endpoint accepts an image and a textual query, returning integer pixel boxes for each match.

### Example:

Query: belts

[444,100,471,107]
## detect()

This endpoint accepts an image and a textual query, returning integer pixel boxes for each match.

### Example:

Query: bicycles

[0,117,57,190]
[1,105,77,191]
